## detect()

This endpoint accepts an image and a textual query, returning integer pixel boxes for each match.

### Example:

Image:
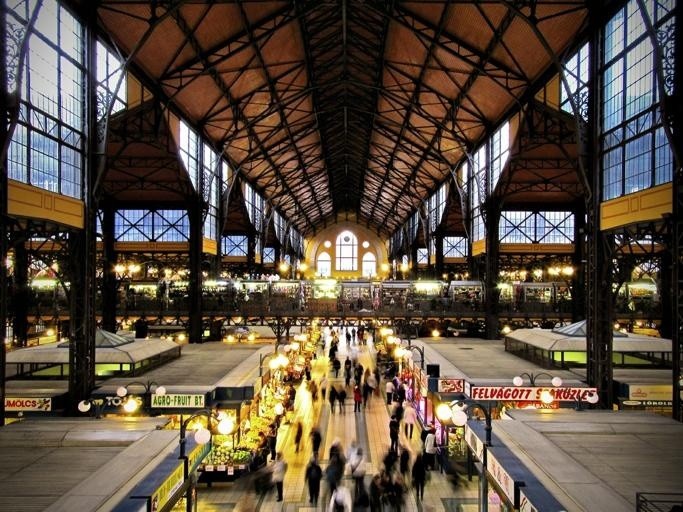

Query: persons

[246,326,457,511]
